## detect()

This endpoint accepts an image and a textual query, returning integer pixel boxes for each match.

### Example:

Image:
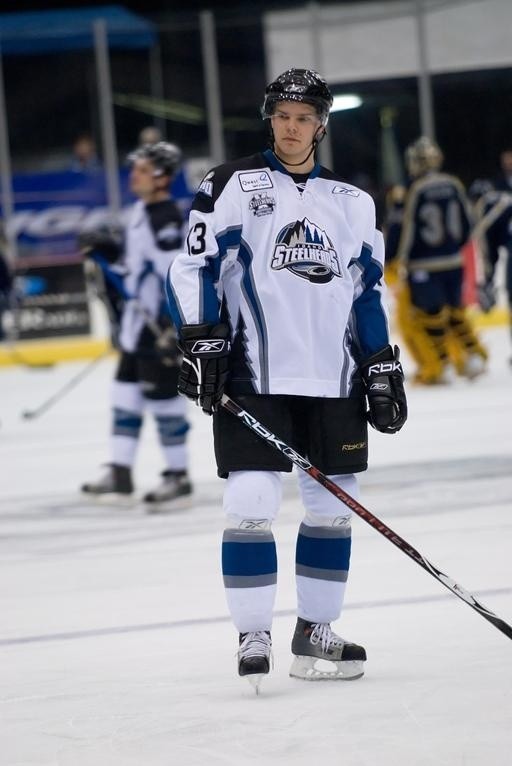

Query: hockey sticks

[21,349,111,420]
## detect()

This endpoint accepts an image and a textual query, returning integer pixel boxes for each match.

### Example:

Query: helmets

[406,134,440,176]
[259,68,332,131]
[126,140,185,192]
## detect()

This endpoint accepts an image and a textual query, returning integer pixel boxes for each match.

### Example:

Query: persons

[163,68,407,674]
[376,133,511,385]
[71,123,197,501]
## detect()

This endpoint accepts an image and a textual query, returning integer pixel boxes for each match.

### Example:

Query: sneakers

[236,630,272,675]
[143,472,193,501]
[292,618,366,662]
[81,463,135,494]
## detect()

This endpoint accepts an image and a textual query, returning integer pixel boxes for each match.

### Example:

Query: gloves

[80,223,124,265]
[176,318,233,413]
[352,343,408,434]
